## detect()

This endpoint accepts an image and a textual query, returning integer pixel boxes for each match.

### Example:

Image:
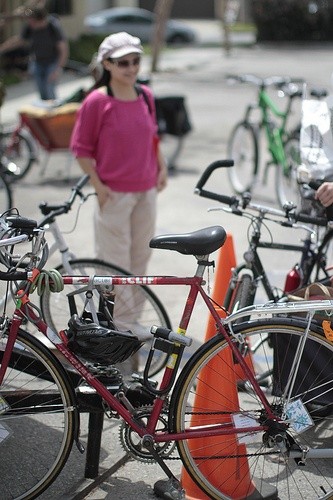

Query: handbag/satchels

[300,84,333,181]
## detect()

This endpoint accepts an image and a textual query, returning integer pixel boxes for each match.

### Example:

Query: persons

[72,32,168,380]
[273,183,333,404]
[0,8,68,102]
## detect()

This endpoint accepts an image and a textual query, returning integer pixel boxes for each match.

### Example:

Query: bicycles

[1,128,333,499]
[222,71,332,212]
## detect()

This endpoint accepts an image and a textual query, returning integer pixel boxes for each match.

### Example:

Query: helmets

[59,312,152,365]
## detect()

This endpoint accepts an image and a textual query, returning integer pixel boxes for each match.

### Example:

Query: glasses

[108,57,141,68]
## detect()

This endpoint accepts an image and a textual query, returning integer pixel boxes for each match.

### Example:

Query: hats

[97,31,144,64]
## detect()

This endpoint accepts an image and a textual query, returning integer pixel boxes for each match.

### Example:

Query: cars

[78,5,197,47]
